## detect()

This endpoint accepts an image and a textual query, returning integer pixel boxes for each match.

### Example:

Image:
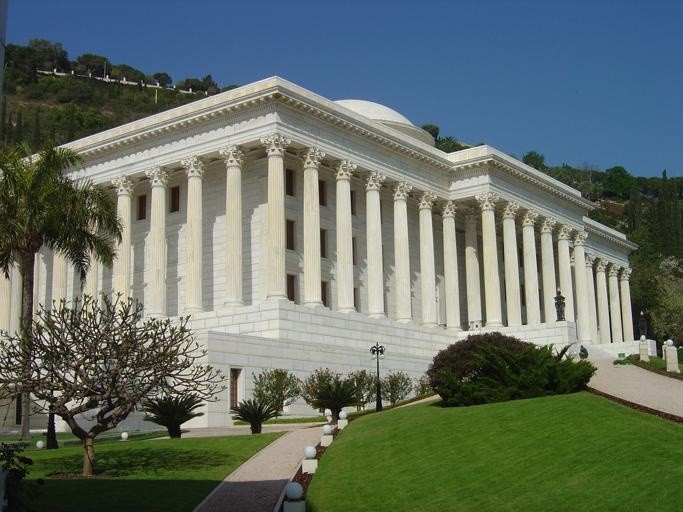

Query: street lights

[640,308,649,337]
[103,61,107,79]
[369,343,387,411]
[554,285,566,321]
